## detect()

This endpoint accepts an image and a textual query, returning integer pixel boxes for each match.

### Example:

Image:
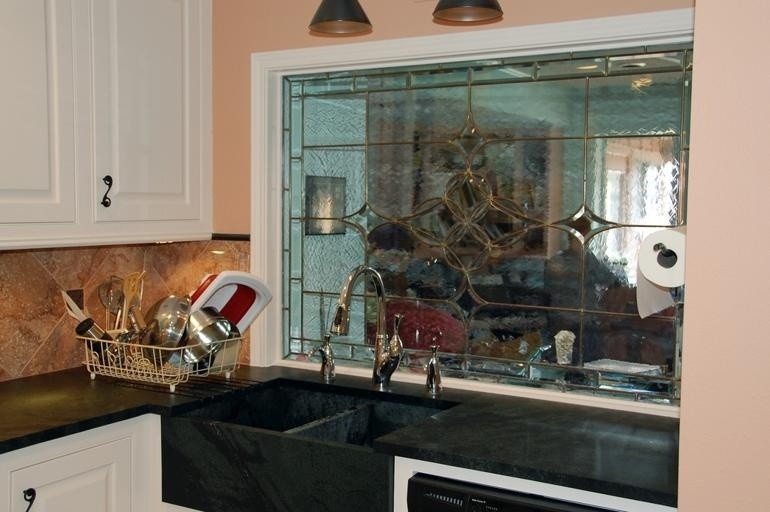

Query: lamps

[431,0,503,26]
[309,0,373,38]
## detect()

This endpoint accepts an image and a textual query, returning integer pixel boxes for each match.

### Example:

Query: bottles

[75,318,118,364]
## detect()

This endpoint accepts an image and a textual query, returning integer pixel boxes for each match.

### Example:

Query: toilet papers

[636,228,686,320]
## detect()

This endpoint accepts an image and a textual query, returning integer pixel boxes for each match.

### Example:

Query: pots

[179,306,231,364]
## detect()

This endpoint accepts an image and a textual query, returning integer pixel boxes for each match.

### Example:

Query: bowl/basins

[138,294,191,368]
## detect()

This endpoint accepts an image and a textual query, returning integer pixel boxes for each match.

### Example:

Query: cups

[554,335,572,364]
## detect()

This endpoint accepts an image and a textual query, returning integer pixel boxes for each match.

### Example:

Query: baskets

[75,328,246,394]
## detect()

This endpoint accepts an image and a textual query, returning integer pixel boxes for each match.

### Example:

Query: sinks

[288,396,461,451]
[175,378,361,433]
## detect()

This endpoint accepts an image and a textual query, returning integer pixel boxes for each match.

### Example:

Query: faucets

[330,268,387,382]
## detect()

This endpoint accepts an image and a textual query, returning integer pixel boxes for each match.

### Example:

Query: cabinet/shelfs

[0,1,213,253]
[0,413,164,511]
